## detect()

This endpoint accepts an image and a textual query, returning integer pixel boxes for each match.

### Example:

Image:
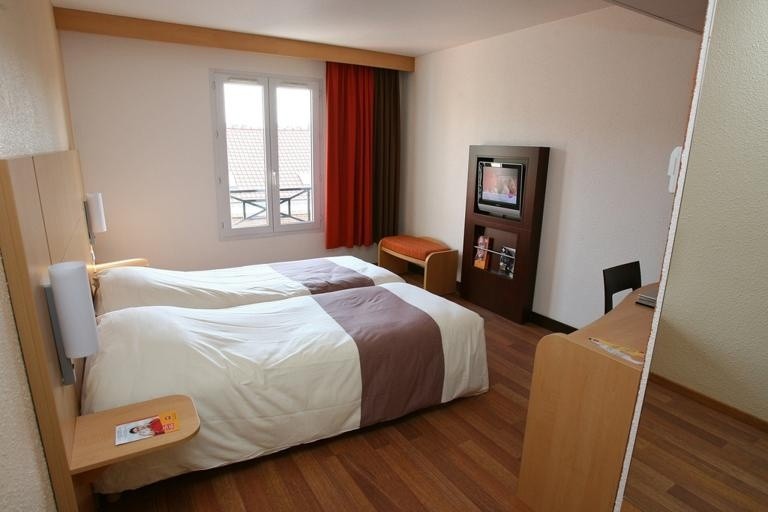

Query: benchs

[375,233,459,296]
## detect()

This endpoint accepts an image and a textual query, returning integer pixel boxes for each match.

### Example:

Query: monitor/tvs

[477,161,524,218]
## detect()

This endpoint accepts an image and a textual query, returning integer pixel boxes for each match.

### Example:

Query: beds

[86,255,488,503]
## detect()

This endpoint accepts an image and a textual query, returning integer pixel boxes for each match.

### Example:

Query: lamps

[40,259,101,385]
[83,191,107,242]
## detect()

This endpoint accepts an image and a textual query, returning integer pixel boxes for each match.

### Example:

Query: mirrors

[615,0,767,511]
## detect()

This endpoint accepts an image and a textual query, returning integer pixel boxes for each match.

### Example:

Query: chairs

[603,262,641,313]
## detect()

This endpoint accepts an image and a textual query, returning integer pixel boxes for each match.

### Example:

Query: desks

[515,280,657,511]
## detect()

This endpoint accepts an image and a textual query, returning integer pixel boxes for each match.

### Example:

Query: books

[498,247,516,279]
[474,236,493,271]
